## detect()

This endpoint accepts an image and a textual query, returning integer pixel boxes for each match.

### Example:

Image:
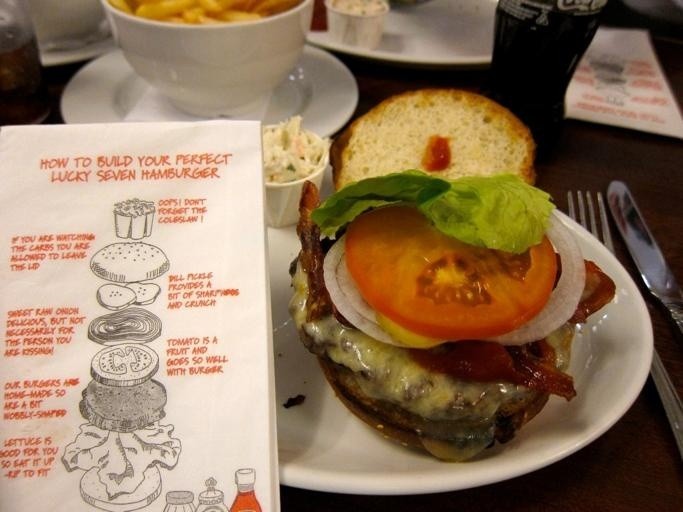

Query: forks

[562,186,683,463]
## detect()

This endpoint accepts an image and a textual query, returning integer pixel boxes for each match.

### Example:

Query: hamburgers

[286,87,617,464]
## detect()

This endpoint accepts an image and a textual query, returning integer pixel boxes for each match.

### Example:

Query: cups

[260,123,329,229]
[98,0,314,120]
[323,1,389,51]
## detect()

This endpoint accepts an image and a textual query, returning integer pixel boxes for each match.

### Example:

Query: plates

[265,167,656,498]
[57,46,361,147]
[25,0,110,68]
[304,1,499,67]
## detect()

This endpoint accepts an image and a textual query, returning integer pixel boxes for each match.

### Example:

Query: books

[0,121,285,511]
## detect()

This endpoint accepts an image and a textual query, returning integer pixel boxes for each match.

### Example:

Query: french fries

[105,0,263,26]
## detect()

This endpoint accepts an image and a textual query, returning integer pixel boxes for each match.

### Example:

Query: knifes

[602,180,683,338]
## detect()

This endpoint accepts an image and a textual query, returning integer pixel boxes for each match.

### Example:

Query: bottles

[229,468,262,512]
[195,487,228,511]
[161,490,195,512]
